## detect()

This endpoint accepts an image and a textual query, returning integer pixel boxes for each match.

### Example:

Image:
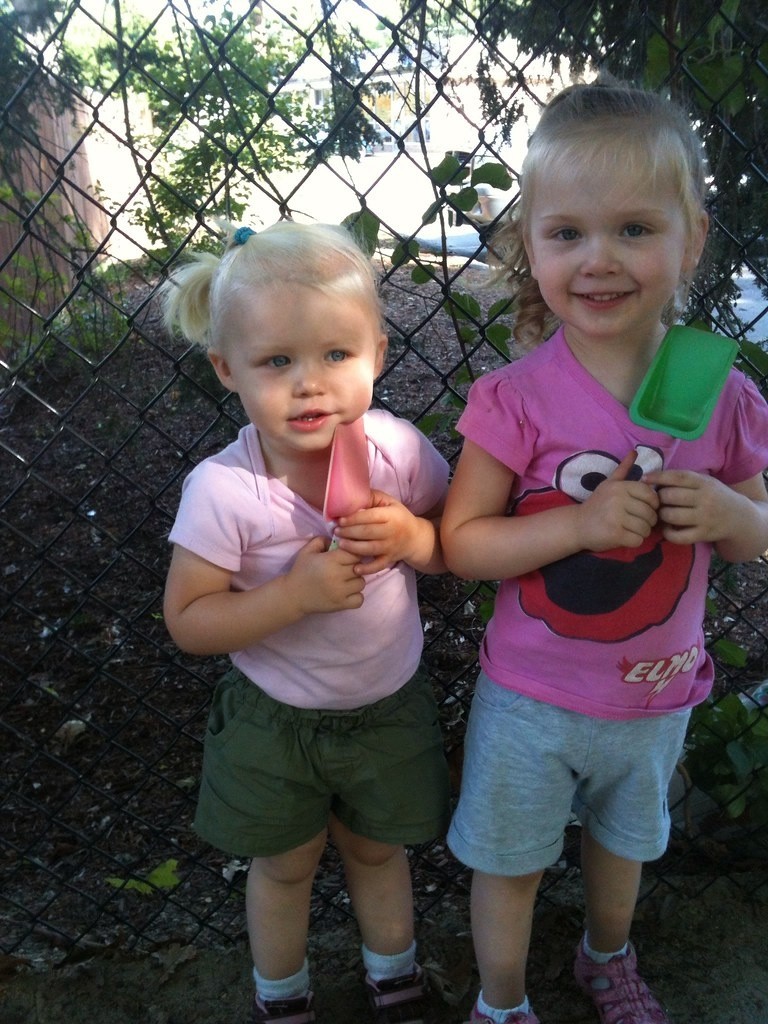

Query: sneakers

[365,961,431,1024]
[253,990,317,1024]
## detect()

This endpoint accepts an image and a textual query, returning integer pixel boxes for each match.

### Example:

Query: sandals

[573,935,669,1024]
[464,1002,541,1024]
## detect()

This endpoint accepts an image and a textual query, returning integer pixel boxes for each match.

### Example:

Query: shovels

[322,414,373,552]
[625,323,741,443]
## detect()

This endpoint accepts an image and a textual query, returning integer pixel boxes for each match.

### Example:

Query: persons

[163,223,456,1024]
[439,85,767,1024]
